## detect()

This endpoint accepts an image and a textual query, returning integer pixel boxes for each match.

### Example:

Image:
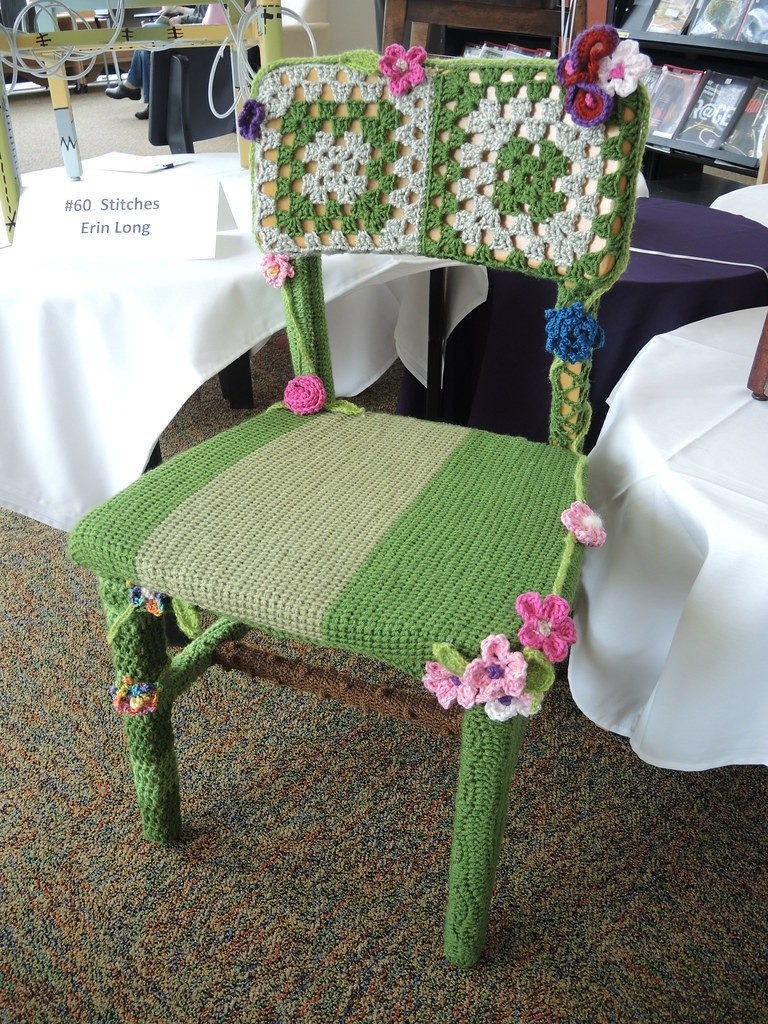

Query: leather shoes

[105,82,142,100]
[134,104,149,119]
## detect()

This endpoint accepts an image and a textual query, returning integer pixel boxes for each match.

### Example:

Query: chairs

[68,54,653,966]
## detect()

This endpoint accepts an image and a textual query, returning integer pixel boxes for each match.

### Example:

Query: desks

[433,199,767,456]
[0,150,490,477]
[566,298,768,771]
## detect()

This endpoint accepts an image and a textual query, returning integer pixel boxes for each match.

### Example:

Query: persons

[105,5,209,120]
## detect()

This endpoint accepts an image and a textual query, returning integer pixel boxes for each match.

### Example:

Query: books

[155,15,170,23]
[638,0,768,158]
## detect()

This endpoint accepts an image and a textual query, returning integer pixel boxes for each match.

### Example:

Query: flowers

[104,29,654,722]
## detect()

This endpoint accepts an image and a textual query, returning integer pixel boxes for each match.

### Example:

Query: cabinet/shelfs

[588,0,768,204]
